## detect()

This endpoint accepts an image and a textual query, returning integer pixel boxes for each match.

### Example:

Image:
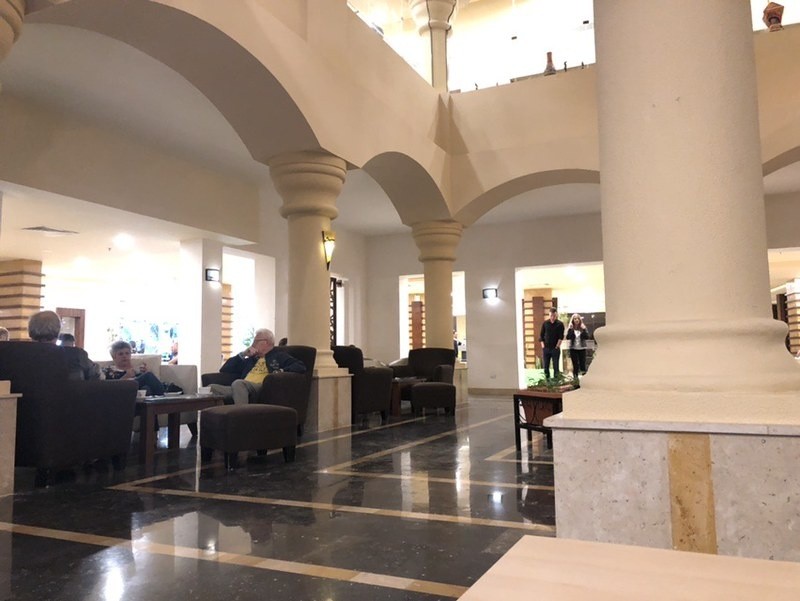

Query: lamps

[482,288,498,299]
[323,230,336,270]
[205,269,221,282]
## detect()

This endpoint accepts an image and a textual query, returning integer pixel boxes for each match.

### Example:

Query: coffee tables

[127,391,226,465]
[392,378,427,417]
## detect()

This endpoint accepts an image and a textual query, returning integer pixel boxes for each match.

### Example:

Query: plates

[137,399,144,403]
[196,392,213,396]
[164,392,179,396]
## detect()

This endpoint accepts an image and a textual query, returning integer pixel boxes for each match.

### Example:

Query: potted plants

[515,369,580,425]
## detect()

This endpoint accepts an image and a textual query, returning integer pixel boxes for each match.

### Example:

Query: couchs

[330,345,394,423]
[200,344,317,458]
[0,340,198,490]
[389,347,456,418]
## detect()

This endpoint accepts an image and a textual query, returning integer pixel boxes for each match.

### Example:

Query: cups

[198,386,211,393]
[137,390,147,399]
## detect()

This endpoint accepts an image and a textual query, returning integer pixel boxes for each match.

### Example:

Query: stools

[410,381,456,418]
[200,404,299,473]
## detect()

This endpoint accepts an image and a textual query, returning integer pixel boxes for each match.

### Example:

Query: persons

[208,329,307,407]
[61,334,75,347]
[101,341,165,396]
[129,339,145,354]
[0,327,9,341]
[168,342,178,365]
[566,314,590,378]
[29,310,106,380]
[453,330,458,356]
[539,307,564,378]
